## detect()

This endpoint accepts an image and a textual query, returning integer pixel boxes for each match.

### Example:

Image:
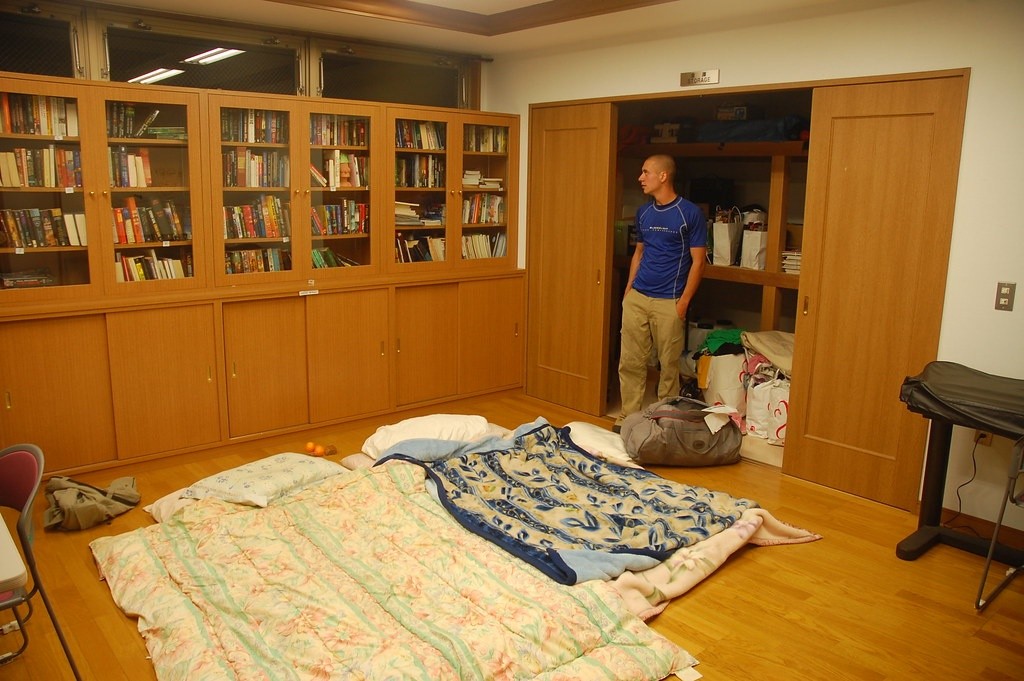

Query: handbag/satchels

[712,203,766,269]
[620,395,746,466]
[690,336,788,451]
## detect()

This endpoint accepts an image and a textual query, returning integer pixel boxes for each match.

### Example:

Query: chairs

[0,444,83,681]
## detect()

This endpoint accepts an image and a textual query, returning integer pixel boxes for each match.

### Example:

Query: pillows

[564,420,644,471]
[360,414,490,461]
[175,452,352,510]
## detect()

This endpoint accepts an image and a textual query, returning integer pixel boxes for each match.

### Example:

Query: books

[224,248,291,274]
[395,120,446,150]
[462,193,503,224]
[0,144,83,188]
[462,233,506,259]
[222,193,291,240]
[395,154,445,188]
[463,126,505,153]
[0,208,88,247]
[108,145,189,188]
[309,149,369,187]
[395,201,446,226]
[311,247,361,268]
[222,147,290,188]
[309,112,368,147]
[0,257,87,288]
[0,91,78,136]
[221,109,288,144]
[462,170,503,188]
[104,101,187,140]
[110,194,191,244]
[115,247,194,283]
[782,249,802,274]
[311,197,369,237]
[395,236,445,263]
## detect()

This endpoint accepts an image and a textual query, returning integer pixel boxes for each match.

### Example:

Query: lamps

[126,45,248,87]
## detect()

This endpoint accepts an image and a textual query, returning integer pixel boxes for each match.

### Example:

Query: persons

[611,153,708,434]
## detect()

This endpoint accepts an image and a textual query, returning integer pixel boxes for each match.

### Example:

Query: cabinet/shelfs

[621,141,809,335]
[0,70,521,479]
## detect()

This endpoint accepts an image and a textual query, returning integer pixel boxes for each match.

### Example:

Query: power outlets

[974,430,993,448]
[994,282,1017,312]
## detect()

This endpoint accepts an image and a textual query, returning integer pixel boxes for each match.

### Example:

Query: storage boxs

[688,320,737,353]
[651,123,698,143]
